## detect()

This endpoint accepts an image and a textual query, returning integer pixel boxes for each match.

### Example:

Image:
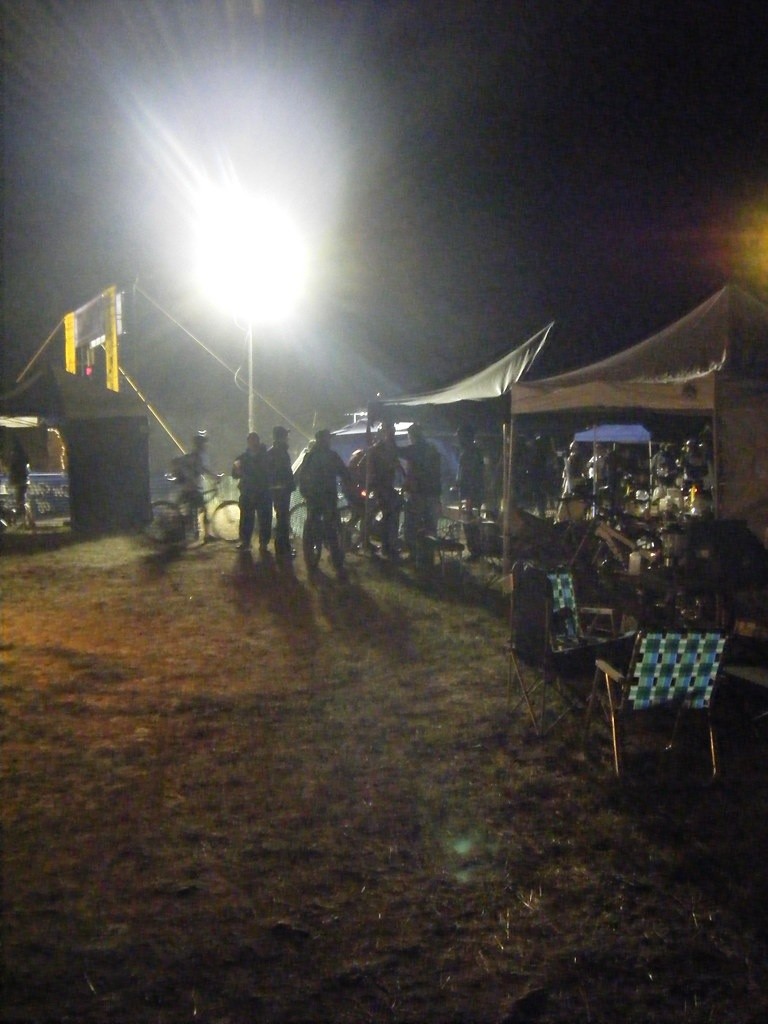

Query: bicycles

[141,472,243,547]
[288,480,411,558]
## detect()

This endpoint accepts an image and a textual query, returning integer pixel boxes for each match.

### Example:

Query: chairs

[502,557,639,742]
[576,624,737,790]
[527,559,627,724]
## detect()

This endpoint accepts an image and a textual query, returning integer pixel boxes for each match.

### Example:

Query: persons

[342,420,767,639]
[173,434,226,542]
[299,430,355,571]
[267,426,297,557]
[7,435,30,520]
[231,431,273,552]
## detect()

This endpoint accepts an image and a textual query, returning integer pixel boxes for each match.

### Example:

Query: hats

[315,429,336,441]
[273,426,289,438]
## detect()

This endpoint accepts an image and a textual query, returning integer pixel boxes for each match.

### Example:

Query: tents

[344,400,368,417]
[291,418,501,500]
[574,423,665,444]
[367,315,640,422]
[507,285,768,411]
[0,361,154,533]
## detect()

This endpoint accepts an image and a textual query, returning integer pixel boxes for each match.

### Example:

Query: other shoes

[236,543,250,551]
[204,533,221,542]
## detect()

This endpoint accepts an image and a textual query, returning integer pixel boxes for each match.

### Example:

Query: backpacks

[171,451,203,483]
